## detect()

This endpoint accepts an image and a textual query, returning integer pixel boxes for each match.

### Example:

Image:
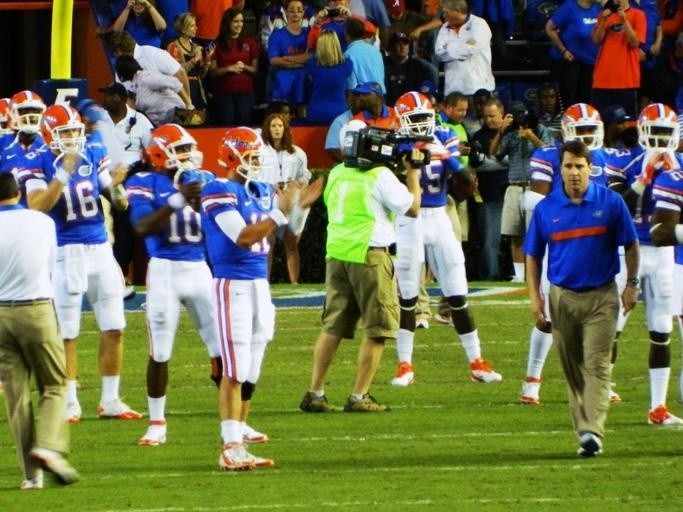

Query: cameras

[604,0,619,13]
[508,113,540,130]
[340,125,435,171]
[326,8,340,17]
[463,141,484,167]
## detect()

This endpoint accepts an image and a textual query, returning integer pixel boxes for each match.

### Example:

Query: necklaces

[178,37,194,54]
[275,148,285,177]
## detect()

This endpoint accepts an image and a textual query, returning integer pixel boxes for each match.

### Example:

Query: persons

[325,82,362,163]
[545,0,602,102]
[650,170,683,398]
[202,126,324,471]
[392,91,503,387]
[628,0,663,70]
[126,123,223,447]
[591,0,646,121]
[350,81,400,131]
[604,104,639,139]
[308,0,376,48]
[213,7,259,126]
[489,104,555,282]
[114,54,194,121]
[109,0,166,48]
[531,83,564,130]
[603,102,682,426]
[0,98,12,129]
[489,0,515,47]
[100,31,190,99]
[382,0,443,39]
[256,114,311,286]
[434,92,470,281]
[1,169,83,490]
[167,12,216,122]
[92,83,154,301]
[463,89,491,135]
[0,91,56,186]
[381,31,439,95]
[299,118,421,413]
[266,0,313,106]
[303,30,357,124]
[522,141,640,457]
[469,97,514,282]
[18,105,143,419]
[343,17,387,104]
[434,0,496,98]
[520,103,618,405]
[76,98,136,300]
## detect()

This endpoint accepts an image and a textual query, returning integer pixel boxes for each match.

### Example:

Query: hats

[386,0,406,13]
[347,81,384,96]
[390,31,409,42]
[97,81,128,97]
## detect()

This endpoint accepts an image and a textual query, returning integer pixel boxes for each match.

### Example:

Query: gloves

[638,151,664,185]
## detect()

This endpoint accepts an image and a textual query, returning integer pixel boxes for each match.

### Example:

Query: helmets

[560,102,605,152]
[636,102,680,153]
[0,96,12,123]
[77,97,107,122]
[216,126,272,183]
[148,122,204,172]
[393,89,436,136]
[39,104,87,155]
[8,90,44,134]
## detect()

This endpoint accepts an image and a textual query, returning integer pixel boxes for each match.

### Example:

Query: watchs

[628,278,640,285]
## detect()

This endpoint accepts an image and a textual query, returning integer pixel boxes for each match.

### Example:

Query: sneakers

[470,358,503,384]
[242,423,268,444]
[217,442,274,472]
[415,313,430,330]
[67,400,83,421]
[26,446,79,483]
[298,391,338,412]
[343,392,391,412]
[434,312,452,324]
[520,378,544,404]
[138,419,168,446]
[390,361,415,386]
[96,398,143,420]
[647,405,683,425]
[20,469,44,489]
[576,433,603,457]
